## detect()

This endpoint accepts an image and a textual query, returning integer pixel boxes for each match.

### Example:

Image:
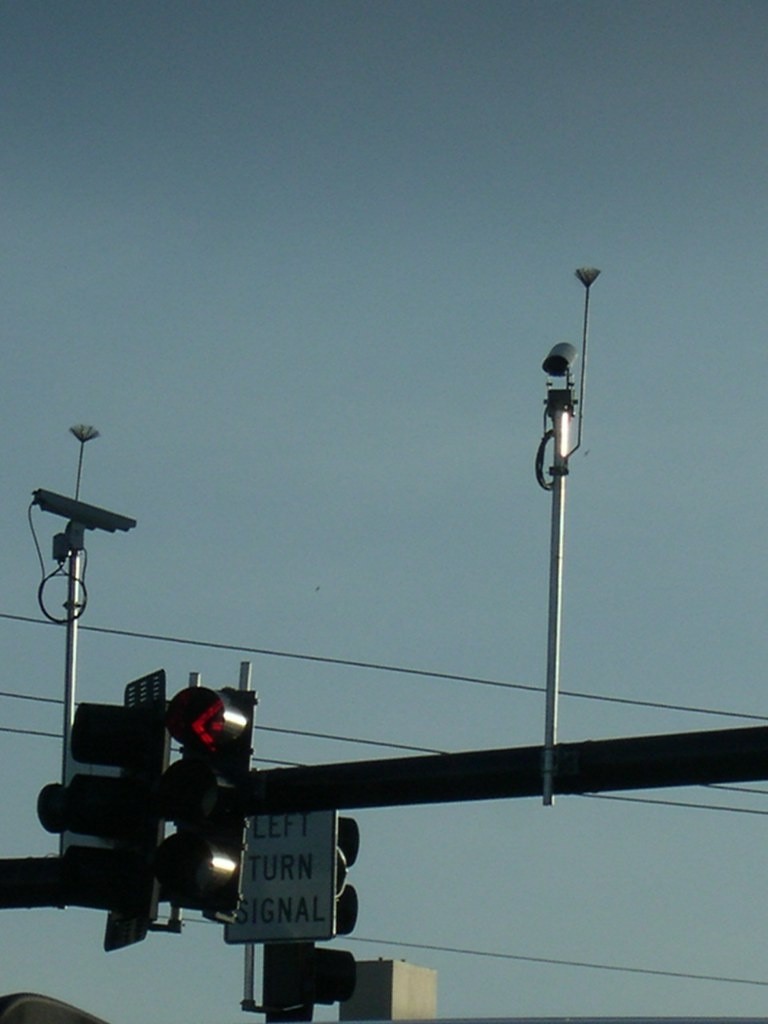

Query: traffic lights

[58,667,170,953]
[152,686,259,915]
[261,816,361,1024]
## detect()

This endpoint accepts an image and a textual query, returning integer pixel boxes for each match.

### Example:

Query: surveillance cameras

[542,341,577,374]
